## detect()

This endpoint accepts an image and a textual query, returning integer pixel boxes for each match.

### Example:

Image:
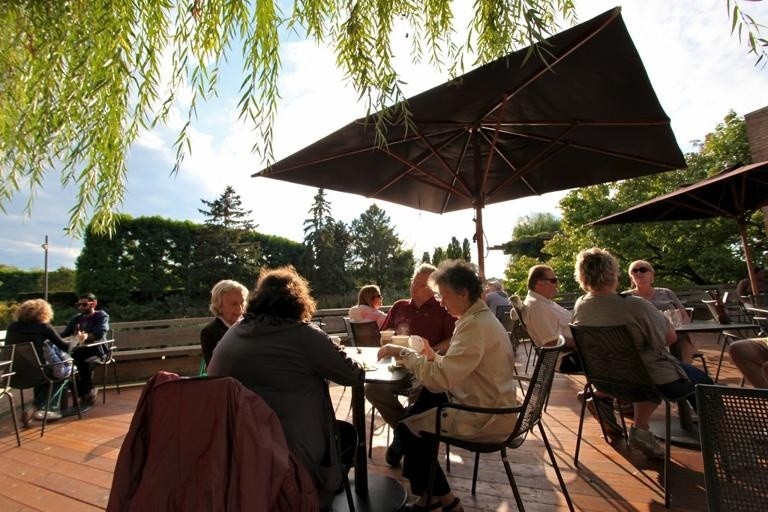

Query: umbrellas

[251,6,688,301]
[581,161,768,311]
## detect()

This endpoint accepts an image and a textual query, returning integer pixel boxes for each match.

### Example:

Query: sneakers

[577,390,590,410]
[21,408,34,427]
[35,411,62,421]
[613,399,634,422]
[586,398,624,436]
[70,403,91,415]
[628,423,664,461]
[87,387,99,404]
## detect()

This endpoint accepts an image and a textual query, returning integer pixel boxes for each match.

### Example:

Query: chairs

[419,335,575,512]
[701,295,759,346]
[156,372,358,512]
[0,344,22,448]
[495,306,517,378]
[566,320,731,508]
[0,342,81,437]
[342,318,382,349]
[93,327,121,404]
[509,295,608,441]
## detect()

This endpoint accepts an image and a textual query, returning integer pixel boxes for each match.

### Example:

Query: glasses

[538,276,558,284]
[373,294,381,299]
[76,301,96,308]
[630,267,655,275]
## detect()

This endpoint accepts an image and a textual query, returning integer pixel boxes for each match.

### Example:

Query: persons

[571,247,730,434]
[4,298,87,420]
[200,280,248,369]
[379,263,457,467]
[349,284,387,331]
[206,271,365,512]
[58,293,110,415]
[620,259,694,366]
[729,337,768,389]
[522,265,634,423]
[486,281,509,316]
[376,266,518,511]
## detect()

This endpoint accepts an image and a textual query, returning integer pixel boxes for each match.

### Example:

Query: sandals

[441,498,465,512]
[403,498,439,512]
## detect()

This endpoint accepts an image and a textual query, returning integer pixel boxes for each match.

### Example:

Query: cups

[380,331,395,346]
[392,336,409,363]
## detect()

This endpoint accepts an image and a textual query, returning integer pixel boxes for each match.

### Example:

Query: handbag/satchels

[42,341,73,380]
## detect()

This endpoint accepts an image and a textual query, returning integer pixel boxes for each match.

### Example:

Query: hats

[487,281,502,290]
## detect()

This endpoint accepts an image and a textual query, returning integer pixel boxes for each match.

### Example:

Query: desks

[329,341,412,512]
[648,316,760,450]
[50,337,115,417]
